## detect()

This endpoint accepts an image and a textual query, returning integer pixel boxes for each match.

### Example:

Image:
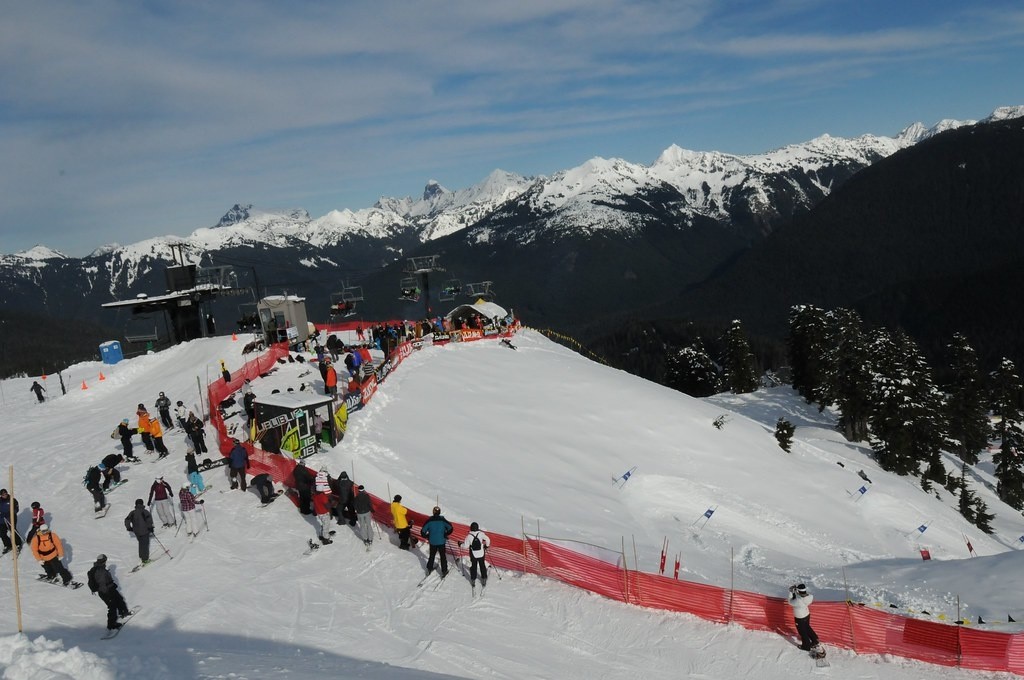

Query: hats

[40,524,49,531]
[433,507,440,515]
[97,463,107,471]
[233,440,240,444]
[189,411,194,416]
[122,419,129,424]
[97,554,107,563]
[341,471,347,478]
[358,485,365,492]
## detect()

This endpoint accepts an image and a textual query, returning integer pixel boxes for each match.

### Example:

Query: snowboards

[219,484,252,494]
[196,457,230,472]
[256,489,284,508]
[103,478,129,495]
[32,573,84,590]
[99,604,142,640]
[193,484,214,500]
[302,528,336,555]
[93,502,112,519]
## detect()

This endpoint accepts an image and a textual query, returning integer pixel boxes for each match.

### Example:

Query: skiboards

[184,519,208,545]
[0,544,23,561]
[176,427,186,434]
[127,548,170,574]
[364,538,373,553]
[149,449,175,464]
[161,424,175,436]
[149,522,175,537]
[416,555,460,591]
[469,564,492,599]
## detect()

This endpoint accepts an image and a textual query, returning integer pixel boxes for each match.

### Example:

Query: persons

[294,459,318,514]
[789,583,820,652]
[0,489,23,553]
[335,471,357,526]
[313,410,324,449]
[311,466,335,519]
[419,507,453,578]
[315,283,444,397]
[389,495,411,550]
[351,485,375,547]
[311,482,332,544]
[23,502,46,546]
[88,554,133,629]
[31,380,47,404]
[456,520,492,587]
[30,522,73,586]
[82,378,280,567]
[268,319,277,344]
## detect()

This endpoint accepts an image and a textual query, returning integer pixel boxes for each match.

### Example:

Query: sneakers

[2,544,22,554]
[107,610,132,630]
[230,480,247,492]
[140,557,152,564]
[261,493,279,503]
[319,535,333,545]
[798,642,821,651]
[124,450,168,462]
[103,481,122,492]
[363,539,373,546]
[428,564,449,578]
[337,519,356,527]
[471,576,487,587]
[95,504,107,515]
[301,508,313,515]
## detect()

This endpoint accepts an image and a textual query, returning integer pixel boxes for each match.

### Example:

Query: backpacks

[468,531,481,550]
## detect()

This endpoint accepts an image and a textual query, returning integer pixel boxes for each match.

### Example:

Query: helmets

[0,489,7,495]
[138,404,144,410]
[177,401,183,406]
[148,415,156,423]
[321,465,327,471]
[155,474,163,483]
[181,481,191,489]
[187,448,195,455]
[159,392,164,396]
[31,502,40,508]
[300,458,306,465]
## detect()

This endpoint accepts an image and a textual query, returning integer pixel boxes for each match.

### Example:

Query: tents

[442,298,508,326]
